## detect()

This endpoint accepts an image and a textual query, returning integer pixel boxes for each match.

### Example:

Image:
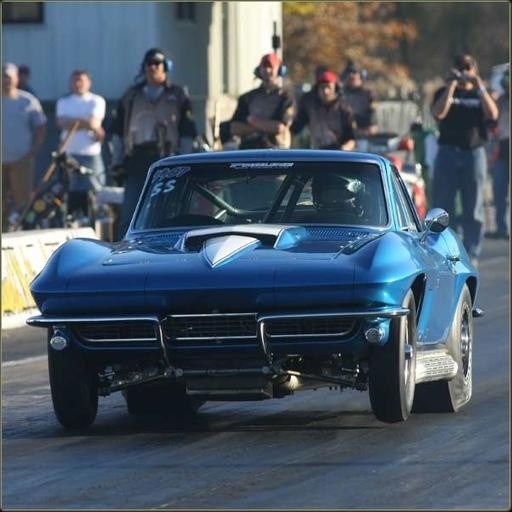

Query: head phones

[254,54,288,79]
[340,63,368,80]
[311,70,344,97]
[139,49,173,74]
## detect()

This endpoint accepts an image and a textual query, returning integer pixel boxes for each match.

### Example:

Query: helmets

[311,171,366,211]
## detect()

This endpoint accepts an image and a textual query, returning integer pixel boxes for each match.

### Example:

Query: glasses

[459,66,471,72]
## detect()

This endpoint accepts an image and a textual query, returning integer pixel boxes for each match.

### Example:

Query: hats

[454,54,475,65]
[143,48,166,64]
[319,70,336,84]
[260,54,281,67]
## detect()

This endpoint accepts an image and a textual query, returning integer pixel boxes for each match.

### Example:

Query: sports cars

[26,148,485,429]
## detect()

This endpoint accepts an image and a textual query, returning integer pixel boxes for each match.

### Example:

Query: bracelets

[478,85,487,97]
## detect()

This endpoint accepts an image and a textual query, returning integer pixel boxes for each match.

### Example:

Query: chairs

[305,184,359,224]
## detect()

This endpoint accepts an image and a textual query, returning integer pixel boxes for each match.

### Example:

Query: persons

[109,48,197,173]
[2,61,50,233]
[338,61,378,153]
[483,63,508,240]
[226,53,297,149]
[55,70,108,222]
[290,69,358,152]
[428,55,499,270]
